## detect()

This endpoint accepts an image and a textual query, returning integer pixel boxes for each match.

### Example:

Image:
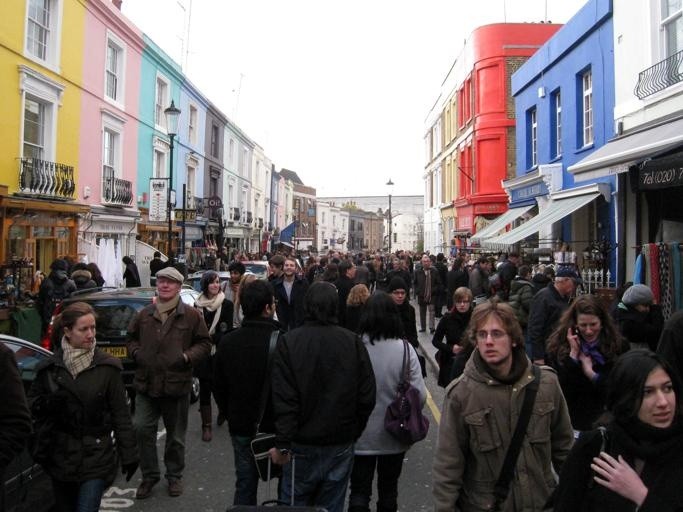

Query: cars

[240,260,270,280]
[190,272,231,290]
[0,334,56,512]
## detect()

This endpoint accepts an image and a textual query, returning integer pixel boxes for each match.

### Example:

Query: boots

[199,405,211,441]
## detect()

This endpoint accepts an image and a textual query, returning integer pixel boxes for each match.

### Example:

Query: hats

[622,285,652,306]
[555,268,582,284]
[156,266,184,282]
[389,278,406,294]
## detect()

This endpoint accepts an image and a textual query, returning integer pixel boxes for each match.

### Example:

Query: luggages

[226,449,329,512]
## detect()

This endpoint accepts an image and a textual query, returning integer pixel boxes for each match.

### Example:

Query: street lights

[383,177,396,251]
[163,98,180,265]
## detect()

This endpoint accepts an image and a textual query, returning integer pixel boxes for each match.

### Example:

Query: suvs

[45,286,215,401]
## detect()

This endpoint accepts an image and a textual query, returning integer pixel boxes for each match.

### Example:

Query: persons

[261,281,376,512]
[128,266,235,500]
[360,291,430,512]
[432,302,577,511]
[34,302,138,512]
[34,254,105,341]
[1,341,39,512]
[224,250,431,378]
[432,250,683,512]
[211,281,284,511]
[150,250,224,288]
[122,256,141,287]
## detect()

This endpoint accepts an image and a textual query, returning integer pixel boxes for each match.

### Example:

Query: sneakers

[136,477,159,499]
[169,479,183,496]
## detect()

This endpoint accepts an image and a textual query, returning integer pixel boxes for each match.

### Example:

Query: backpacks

[41,277,66,321]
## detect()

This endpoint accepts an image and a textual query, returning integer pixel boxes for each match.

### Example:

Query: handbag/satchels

[252,435,283,481]
[385,382,429,443]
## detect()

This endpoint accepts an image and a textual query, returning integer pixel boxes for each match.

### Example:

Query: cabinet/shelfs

[2,263,34,307]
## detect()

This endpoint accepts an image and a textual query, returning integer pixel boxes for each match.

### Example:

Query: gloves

[122,462,140,482]
